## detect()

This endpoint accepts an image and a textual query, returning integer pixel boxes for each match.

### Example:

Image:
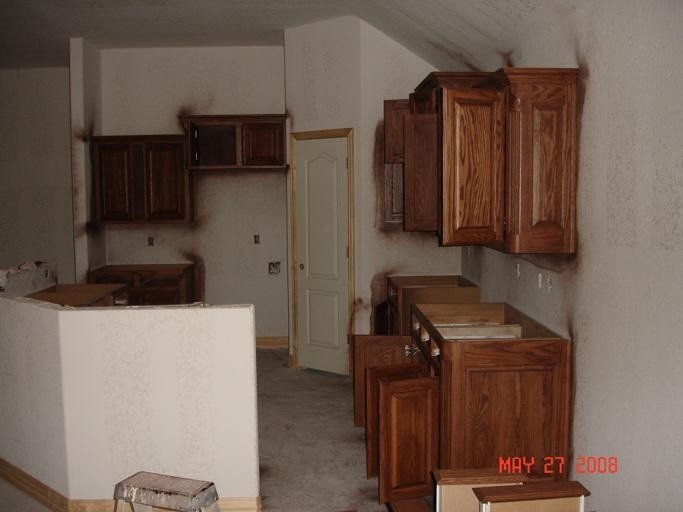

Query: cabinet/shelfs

[87,121,284,222]
[91,274,203,305]
[353,277,570,503]
[381,82,575,254]
